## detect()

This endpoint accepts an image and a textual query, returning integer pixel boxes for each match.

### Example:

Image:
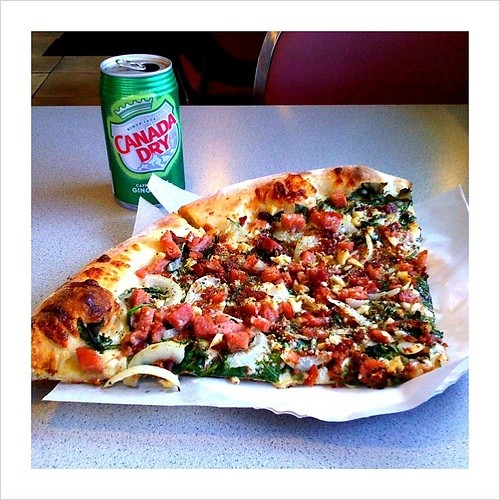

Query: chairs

[177,31,469,106]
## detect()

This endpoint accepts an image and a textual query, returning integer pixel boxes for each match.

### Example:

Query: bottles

[98,54,186,211]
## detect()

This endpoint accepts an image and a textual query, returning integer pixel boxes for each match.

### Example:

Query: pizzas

[31,163,448,387]
